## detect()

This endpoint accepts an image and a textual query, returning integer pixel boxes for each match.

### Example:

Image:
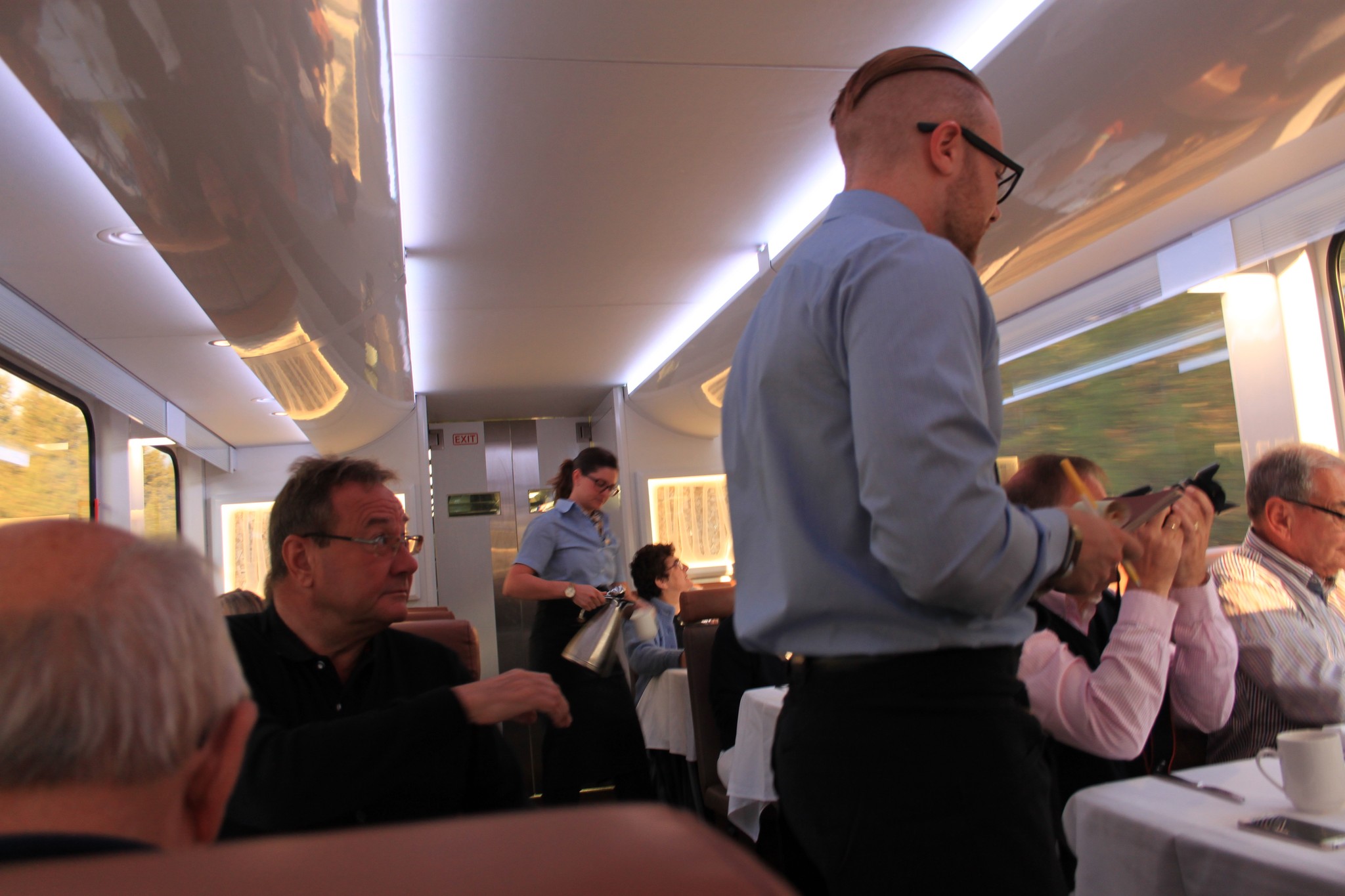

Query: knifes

[1150,770,1245,804]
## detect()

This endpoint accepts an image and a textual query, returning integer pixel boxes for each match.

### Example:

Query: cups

[1255,729,1345,815]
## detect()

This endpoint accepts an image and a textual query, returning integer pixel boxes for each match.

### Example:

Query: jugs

[561,586,637,677]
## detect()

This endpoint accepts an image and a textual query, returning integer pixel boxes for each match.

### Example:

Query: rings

[1166,522,1177,530]
[1193,521,1200,532]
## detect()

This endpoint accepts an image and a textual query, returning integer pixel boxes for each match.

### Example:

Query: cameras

[1162,462,1242,528]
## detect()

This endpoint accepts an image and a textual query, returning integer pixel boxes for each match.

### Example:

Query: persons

[1007,442,1345,896]
[719,47,1153,896]
[0,446,805,867]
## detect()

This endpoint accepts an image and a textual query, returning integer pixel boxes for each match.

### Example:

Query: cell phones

[1238,815,1345,853]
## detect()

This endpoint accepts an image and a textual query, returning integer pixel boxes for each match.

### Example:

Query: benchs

[0,606,791,896]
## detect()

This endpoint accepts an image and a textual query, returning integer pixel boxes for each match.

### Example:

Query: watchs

[564,582,578,599]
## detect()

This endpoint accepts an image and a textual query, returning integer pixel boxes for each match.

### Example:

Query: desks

[1062,758,1345,896]
[637,668,699,817]
[717,685,778,845]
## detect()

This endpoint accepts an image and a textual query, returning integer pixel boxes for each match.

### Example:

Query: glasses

[660,559,683,573]
[581,470,620,497]
[917,121,1024,204]
[299,532,423,557]
